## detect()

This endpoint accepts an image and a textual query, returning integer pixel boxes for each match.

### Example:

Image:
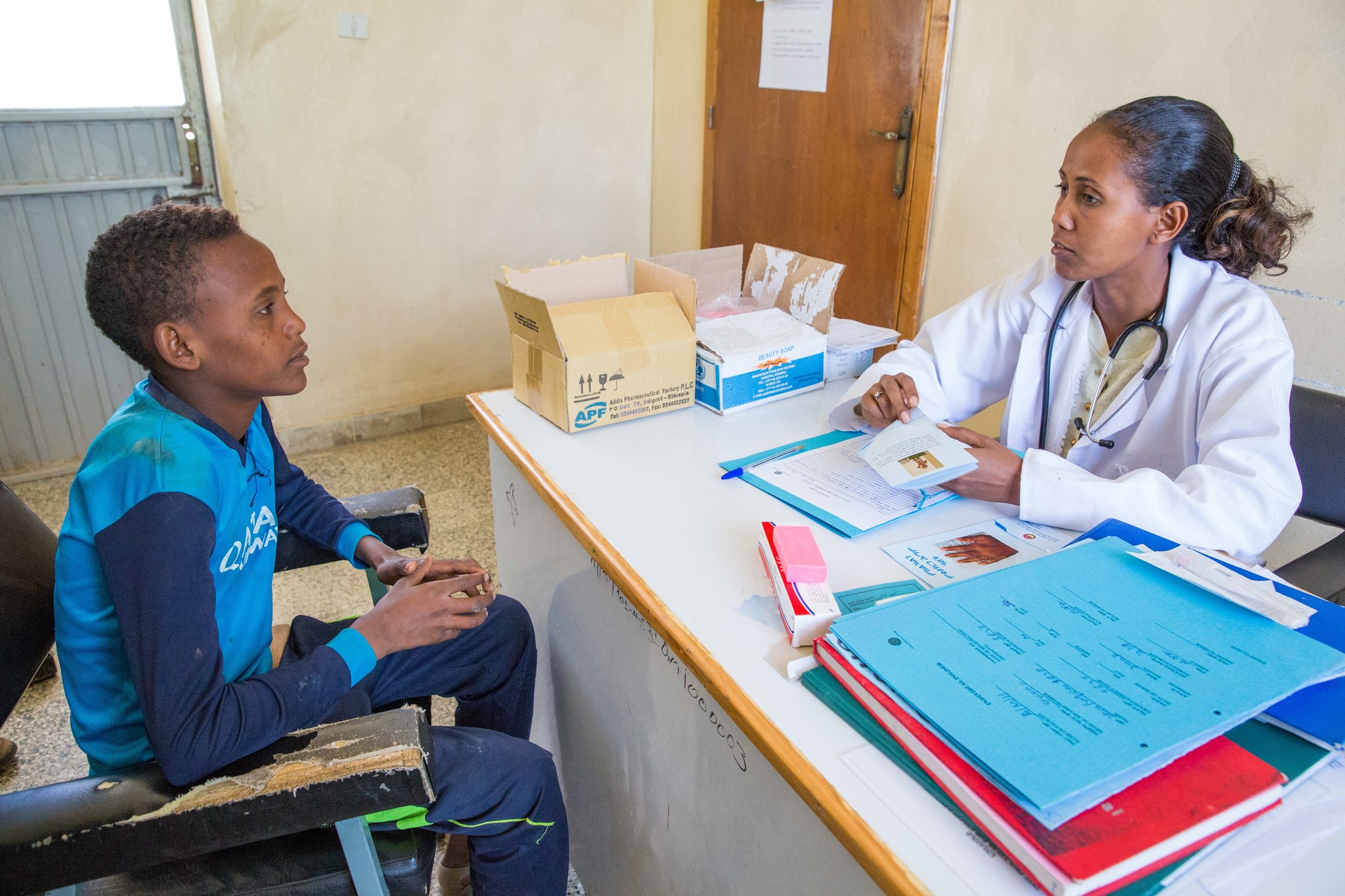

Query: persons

[825,97,1314,558]
[54,203,569,896]
[940,535,1019,565]
[912,457,928,469]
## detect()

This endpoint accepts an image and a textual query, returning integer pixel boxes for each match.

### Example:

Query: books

[813,633,1292,896]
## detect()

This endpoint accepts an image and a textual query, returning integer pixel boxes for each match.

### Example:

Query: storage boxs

[757,521,841,648]
[493,242,874,434]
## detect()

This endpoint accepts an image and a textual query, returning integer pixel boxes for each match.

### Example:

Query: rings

[874,391,887,401]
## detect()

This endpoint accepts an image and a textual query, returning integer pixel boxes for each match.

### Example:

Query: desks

[465,375,1345,896]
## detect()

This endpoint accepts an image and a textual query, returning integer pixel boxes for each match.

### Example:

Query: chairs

[1271,384,1342,606]
[0,489,433,895]
